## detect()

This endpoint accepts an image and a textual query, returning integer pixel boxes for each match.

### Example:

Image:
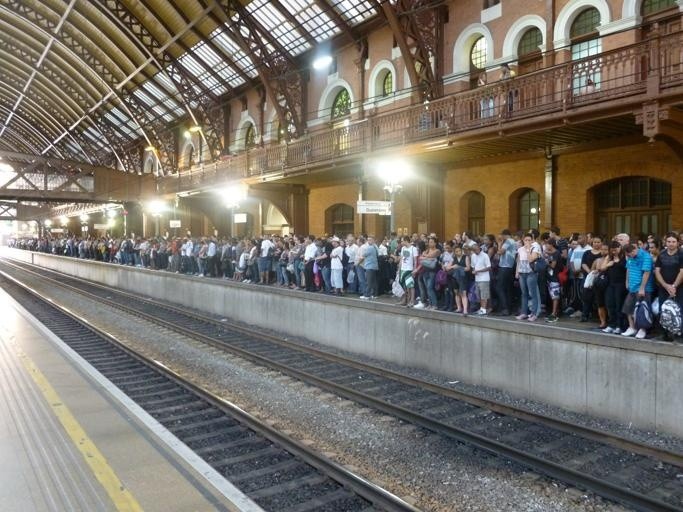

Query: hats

[500,230,510,235]
[330,237,340,242]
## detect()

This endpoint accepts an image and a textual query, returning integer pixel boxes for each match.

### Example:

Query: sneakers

[473,306,492,315]
[601,326,647,339]
[562,307,584,318]
[516,308,559,323]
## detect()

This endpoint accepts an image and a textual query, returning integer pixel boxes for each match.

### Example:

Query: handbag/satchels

[530,247,548,273]
[435,270,448,285]
[346,269,355,283]
[313,258,318,273]
[420,258,437,269]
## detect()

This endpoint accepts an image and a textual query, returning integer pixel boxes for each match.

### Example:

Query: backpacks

[338,249,349,265]
[659,297,682,336]
[634,296,654,328]
[558,266,568,284]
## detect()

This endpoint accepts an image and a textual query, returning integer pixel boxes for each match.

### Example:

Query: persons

[498,63,517,112]
[368,101,380,140]
[582,79,594,95]
[290,126,312,142]
[477,70,494,118]
